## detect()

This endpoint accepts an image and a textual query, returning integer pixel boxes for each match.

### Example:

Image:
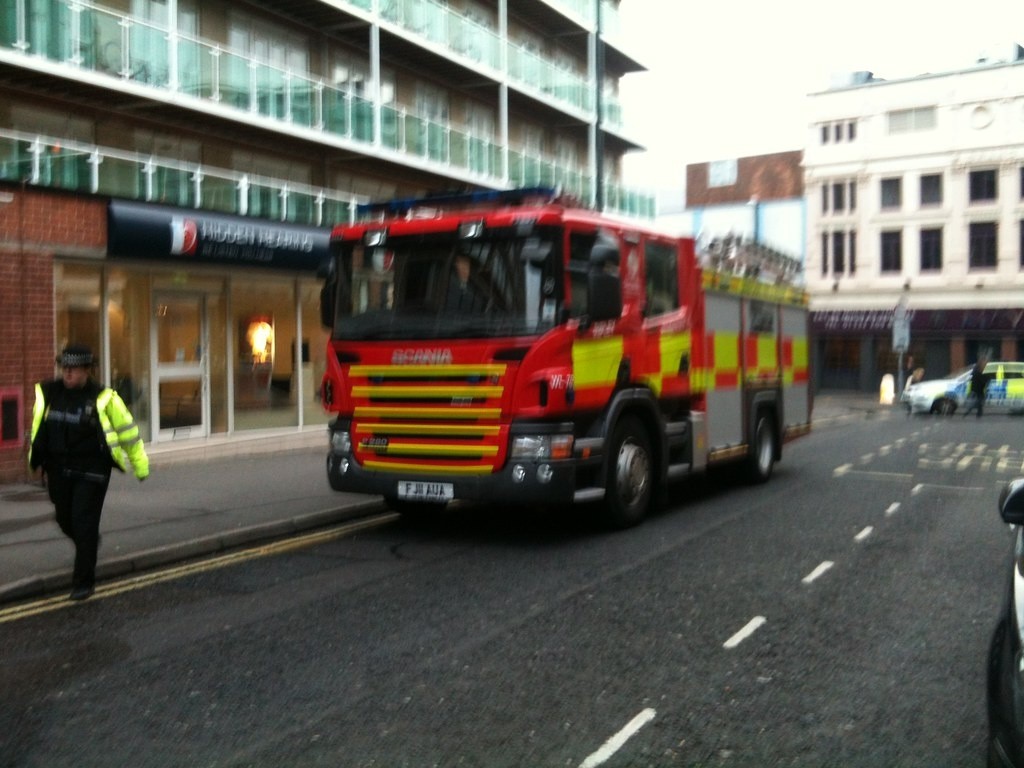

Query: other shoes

[70,586,94,600]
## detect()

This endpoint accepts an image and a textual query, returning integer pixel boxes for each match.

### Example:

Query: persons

[27,342,151,600]
[904,367,926,387]
[904,357,914,377]
[960,359,991,418]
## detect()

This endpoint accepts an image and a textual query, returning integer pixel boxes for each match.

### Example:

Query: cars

[983,467,1024,768]
[896,361,1023,416]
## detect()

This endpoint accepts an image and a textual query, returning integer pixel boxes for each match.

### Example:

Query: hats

[55,344,93,368]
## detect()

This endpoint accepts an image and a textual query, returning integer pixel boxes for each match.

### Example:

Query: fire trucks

[307,183,816,527]
